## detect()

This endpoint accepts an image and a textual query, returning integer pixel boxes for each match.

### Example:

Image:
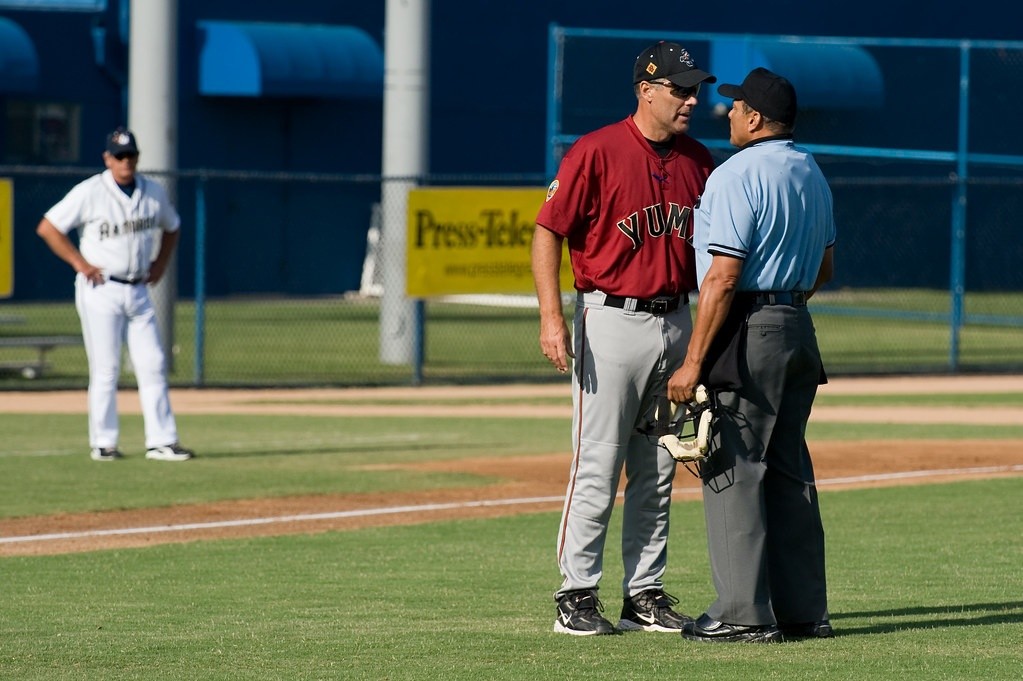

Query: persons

[666,67,836,643]
[530,41,720,637]
[38,131,192,460]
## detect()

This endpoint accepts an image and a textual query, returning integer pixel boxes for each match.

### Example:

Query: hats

[107,128,137,155]
[633,42,716,87]
[718,68,798,123]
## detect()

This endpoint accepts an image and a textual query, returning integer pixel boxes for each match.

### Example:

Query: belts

[733,291,808,307]
[109,276,144,285]
[604,293,690,317]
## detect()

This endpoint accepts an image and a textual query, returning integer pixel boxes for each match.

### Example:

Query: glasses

[648,82,701,100]
[112,152,136,160]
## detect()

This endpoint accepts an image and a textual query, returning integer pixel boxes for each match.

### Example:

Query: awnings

[0,17,37,93]
[196,19,384,97]
[709,35,887,111]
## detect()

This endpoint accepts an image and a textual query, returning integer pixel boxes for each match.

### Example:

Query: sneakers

[145,444,192,461]
[554,588,613,636]
[90,447,121,461]
[616,589,695,633]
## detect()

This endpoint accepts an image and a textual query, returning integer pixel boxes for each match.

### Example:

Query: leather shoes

[681,613,779,643]
[781,619,832,637]
